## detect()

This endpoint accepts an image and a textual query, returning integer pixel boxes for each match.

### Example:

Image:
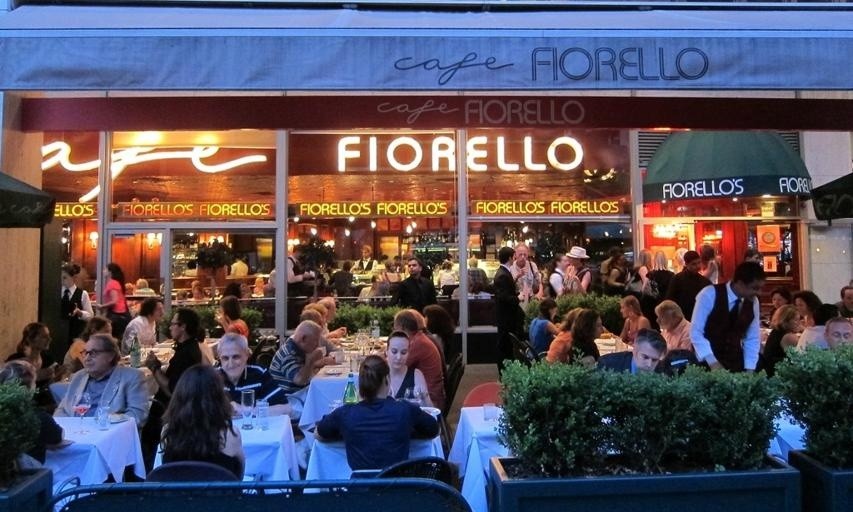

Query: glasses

[80,350,104,357]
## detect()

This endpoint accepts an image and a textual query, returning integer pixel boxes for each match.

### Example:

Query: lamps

[89,232,98,248]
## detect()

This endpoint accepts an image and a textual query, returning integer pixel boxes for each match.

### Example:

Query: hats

[566,246,590,259]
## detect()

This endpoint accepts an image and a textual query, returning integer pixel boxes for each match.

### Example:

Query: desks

[40,318,464,512]
[88,260,500,318]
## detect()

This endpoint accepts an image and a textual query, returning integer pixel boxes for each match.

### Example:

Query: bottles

[129,331,140,368]
[480,232,486,248]
[421,231,455,243]
[503,227,533,248]
[204,329,209,342]
[342,373,357,403]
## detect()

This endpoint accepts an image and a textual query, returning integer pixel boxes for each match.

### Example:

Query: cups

[615,338,628,353]
[97,400,110,430]
[240,388,255,429]
[348,355,360,373]
[334,351,346,362]
[255,397,269,432]
[402,387,420,403]
[354,319,380,355]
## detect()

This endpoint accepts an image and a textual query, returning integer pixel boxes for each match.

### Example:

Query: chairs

[508,312,773,382]
[464,382,504,406]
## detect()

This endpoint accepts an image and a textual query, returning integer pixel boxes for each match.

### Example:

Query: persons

[1,243,853,490]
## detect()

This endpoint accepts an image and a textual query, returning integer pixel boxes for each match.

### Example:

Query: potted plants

[775,343,853,512]
[0,378,52,512]
[489,358,801,512]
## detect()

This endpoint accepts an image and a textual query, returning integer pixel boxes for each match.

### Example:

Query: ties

[730,300,740,319]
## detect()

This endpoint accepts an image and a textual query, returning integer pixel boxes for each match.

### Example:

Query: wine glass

[73,392,91,435]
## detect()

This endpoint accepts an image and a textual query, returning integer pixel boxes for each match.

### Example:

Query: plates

[420,406,441,416]
[324,370,341,374]
[603,339,616,345]
